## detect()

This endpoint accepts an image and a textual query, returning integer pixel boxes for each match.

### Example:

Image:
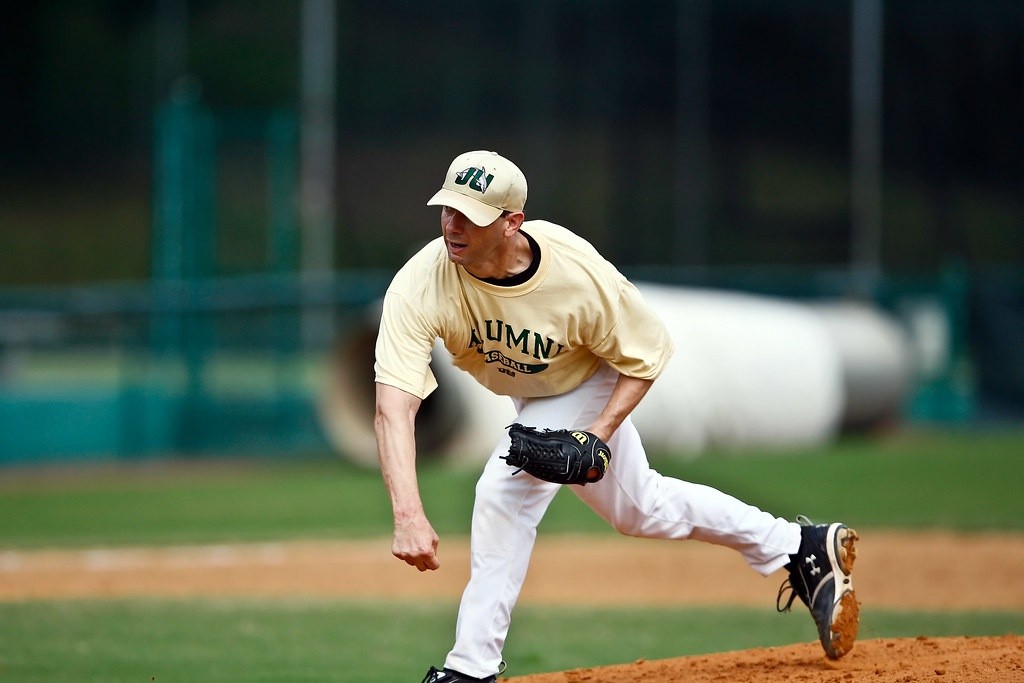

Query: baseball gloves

[498,422,612,487]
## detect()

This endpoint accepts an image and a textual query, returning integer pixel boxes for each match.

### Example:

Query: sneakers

[420,659,507,683]
[775,514,859,660]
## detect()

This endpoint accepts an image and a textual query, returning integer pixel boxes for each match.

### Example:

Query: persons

[370,149,862,682]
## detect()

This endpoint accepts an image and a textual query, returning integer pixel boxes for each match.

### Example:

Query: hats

[427,149,527,227]
